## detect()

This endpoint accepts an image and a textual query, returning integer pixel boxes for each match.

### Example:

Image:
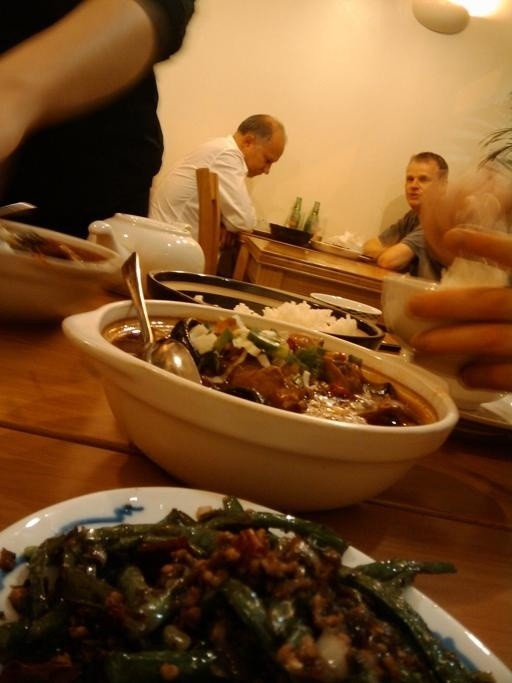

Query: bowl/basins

[58,300,460,516]
[268,221,313,244]
[309,292,385,325]
[146,268,385,352]
[382,273,483,372]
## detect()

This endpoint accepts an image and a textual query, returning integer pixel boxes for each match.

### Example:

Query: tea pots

[84,211,205,297]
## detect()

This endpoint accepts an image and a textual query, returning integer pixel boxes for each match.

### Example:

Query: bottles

[305,199,321,233]
[287,196,304,230]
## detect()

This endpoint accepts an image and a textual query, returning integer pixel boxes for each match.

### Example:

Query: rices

[227,297,362,337]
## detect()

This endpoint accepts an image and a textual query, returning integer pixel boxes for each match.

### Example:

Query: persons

[363,154,450,283]
[407,197,512,391]
[152,113,286,261]
[1,0,195,237]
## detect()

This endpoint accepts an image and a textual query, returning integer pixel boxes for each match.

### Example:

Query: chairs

[196,167,221,275]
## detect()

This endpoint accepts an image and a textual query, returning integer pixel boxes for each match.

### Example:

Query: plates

[310,237,363,259]
[0,483,512,682]
[454,387,512,436]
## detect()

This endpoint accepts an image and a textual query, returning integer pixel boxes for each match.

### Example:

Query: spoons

[120,250,201,385]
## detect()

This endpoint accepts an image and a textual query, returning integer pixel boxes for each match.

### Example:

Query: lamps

[412,1,500,34]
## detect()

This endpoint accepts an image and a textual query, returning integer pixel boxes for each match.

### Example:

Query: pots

[0,219,126,319]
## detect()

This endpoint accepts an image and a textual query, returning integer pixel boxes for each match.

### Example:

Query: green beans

[0,493,494,683]
